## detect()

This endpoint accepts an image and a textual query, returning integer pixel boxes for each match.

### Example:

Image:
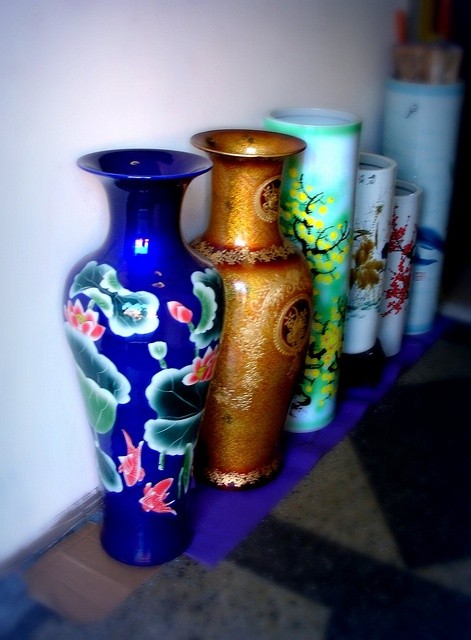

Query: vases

[188,128,313,490]
[63,149,226,568]
